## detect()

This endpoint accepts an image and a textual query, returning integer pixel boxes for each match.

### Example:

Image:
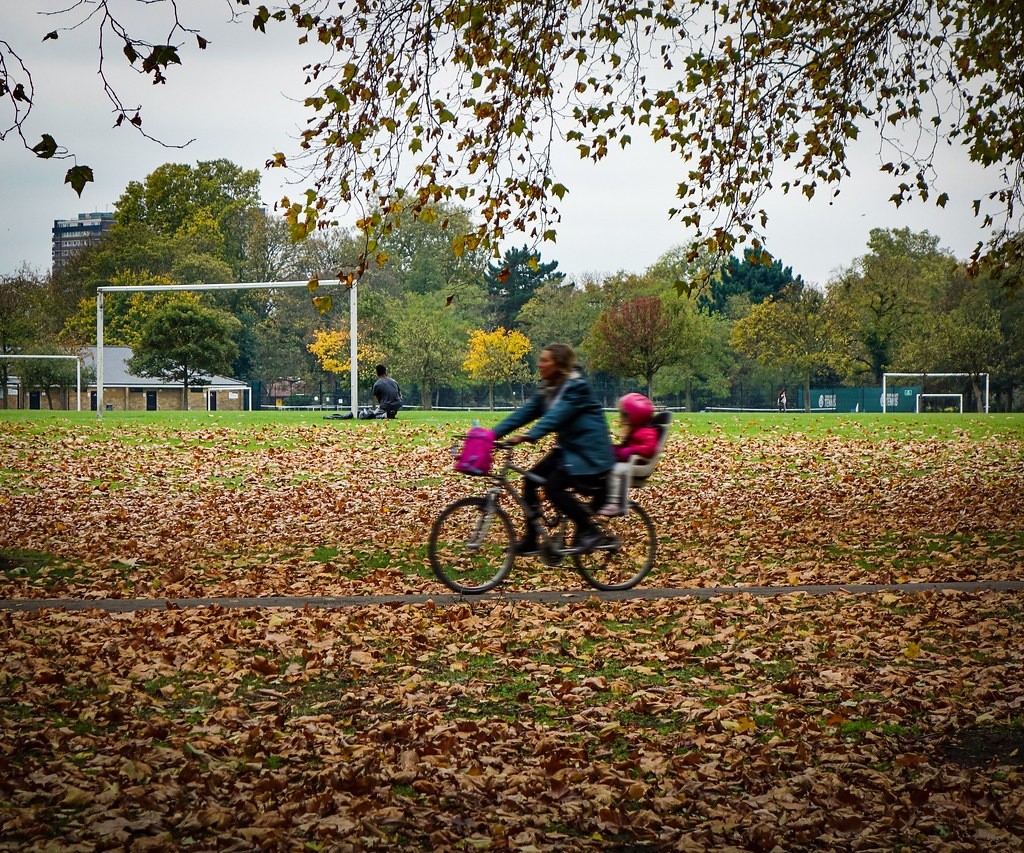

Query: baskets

[451,434,503,477]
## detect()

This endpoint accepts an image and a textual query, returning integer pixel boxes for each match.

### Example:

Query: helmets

[620,392,654,427]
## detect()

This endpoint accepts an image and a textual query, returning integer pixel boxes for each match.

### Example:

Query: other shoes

[567,527,600,554]
[504,536,539,553]
[598,504,622,516]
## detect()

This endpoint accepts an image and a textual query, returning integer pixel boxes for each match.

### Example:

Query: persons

[490,344,615,554]
[371,364,403,419]
[777,389,787,412]
[600,393,660,515]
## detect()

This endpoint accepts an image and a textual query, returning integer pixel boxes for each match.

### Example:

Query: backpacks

[455,420,495,475]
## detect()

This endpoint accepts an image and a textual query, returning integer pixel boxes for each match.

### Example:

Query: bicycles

[429,433,658,592]
